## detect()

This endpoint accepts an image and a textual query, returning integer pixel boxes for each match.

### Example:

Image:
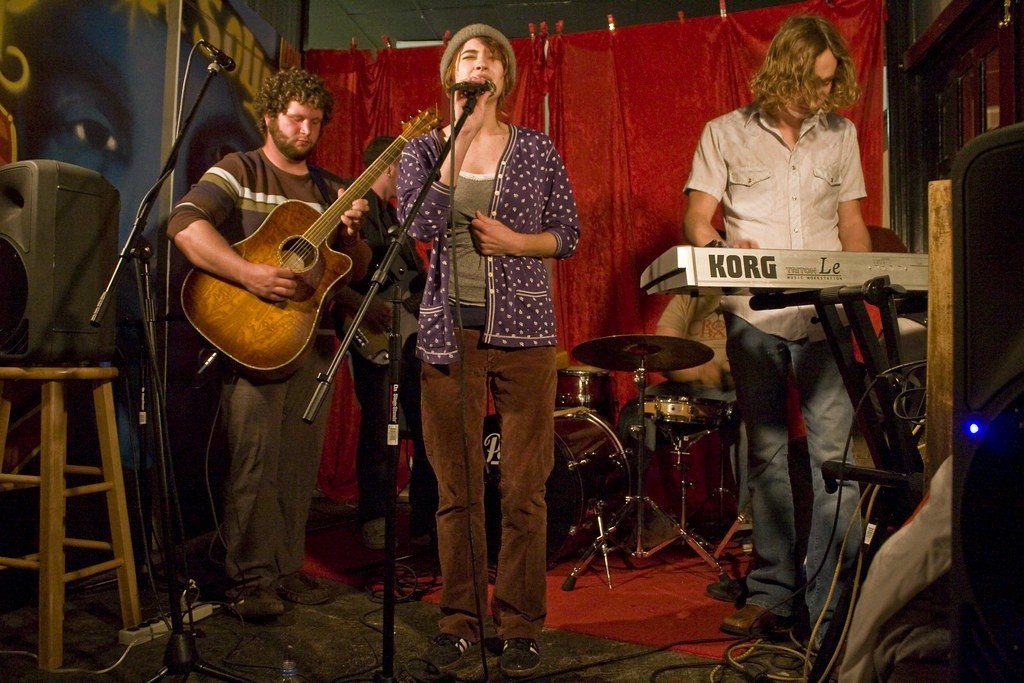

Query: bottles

[273,660,305,683]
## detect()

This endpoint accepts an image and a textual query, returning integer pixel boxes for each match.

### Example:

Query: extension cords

[120,602,211,646]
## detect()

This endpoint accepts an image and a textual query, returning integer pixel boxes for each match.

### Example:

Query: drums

[643,394,735,435]
[483,407,630,585]
[556,366,616,425]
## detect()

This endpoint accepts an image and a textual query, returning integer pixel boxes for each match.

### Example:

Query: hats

[439,23,516,102]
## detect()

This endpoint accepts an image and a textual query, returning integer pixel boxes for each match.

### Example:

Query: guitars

[335,271,419,365]
[179,107,444,382]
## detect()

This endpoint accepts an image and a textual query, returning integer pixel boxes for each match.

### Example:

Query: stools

[0,366,143,671]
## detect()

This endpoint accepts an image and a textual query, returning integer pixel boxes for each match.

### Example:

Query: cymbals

[571,333,716,373]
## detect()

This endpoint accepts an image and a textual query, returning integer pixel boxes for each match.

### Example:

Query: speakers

[947,124,1023,683]
[0,160,121,364]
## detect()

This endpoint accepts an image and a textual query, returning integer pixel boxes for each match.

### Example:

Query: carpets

[299,506,759,663]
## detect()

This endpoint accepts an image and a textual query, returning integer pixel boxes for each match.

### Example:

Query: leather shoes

[720,604,797,635]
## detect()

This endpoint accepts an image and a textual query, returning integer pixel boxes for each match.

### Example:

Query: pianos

[640,245,927,471]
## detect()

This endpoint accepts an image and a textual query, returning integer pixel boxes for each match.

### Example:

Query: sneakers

[500,639,542,677]
[425,632,484,669]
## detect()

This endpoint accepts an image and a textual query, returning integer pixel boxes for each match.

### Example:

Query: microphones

[200,39,236,71]
[451,80,496,93]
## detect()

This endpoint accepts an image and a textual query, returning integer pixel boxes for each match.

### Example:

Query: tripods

[88,59,256,683]
[561,352,732,592]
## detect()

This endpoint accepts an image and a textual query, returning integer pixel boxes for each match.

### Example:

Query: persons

[681,16,872,658]
[398,24,580,677]
[342,136,436,546]
[163,67,369,618]
[707,311,928,602]
[618,291,753,556]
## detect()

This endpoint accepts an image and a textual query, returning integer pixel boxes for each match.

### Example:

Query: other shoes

[274,570,338,604]
[741,528,753,553]
[221,583,285,619]
